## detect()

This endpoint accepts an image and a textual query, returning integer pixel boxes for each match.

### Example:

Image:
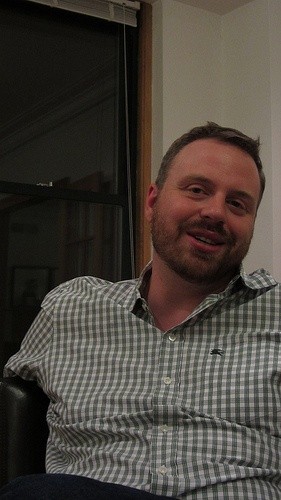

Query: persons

[3,121,281,500]
